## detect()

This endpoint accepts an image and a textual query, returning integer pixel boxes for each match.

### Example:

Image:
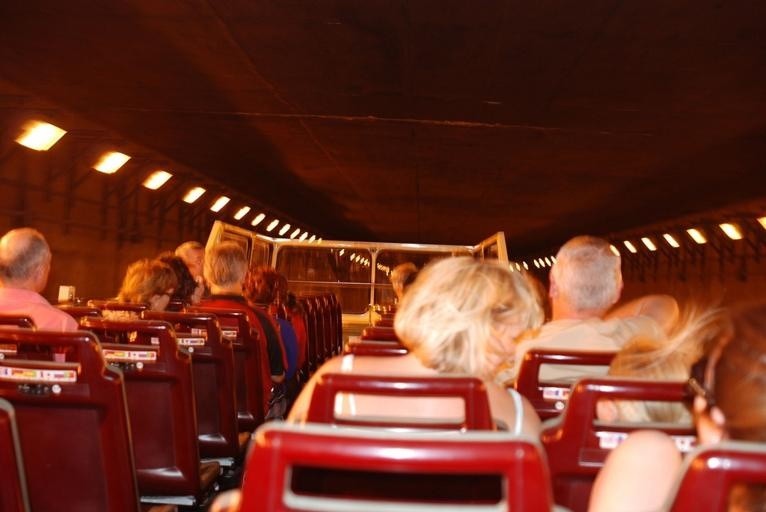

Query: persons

[581,308,698,452]
[207,255,573,512]
[153,238,308,390]
[525,235,683,387]
[389,262,420,301]
[584,298,766,512]
[96,258,177,342]
[0,227,78,362]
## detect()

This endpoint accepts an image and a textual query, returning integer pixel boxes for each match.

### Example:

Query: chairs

[0,291,766,512]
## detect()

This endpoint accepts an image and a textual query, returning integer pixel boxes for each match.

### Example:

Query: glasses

[153,291,176,299]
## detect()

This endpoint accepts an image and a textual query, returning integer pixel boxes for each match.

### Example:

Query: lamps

[507,211,766,272]
